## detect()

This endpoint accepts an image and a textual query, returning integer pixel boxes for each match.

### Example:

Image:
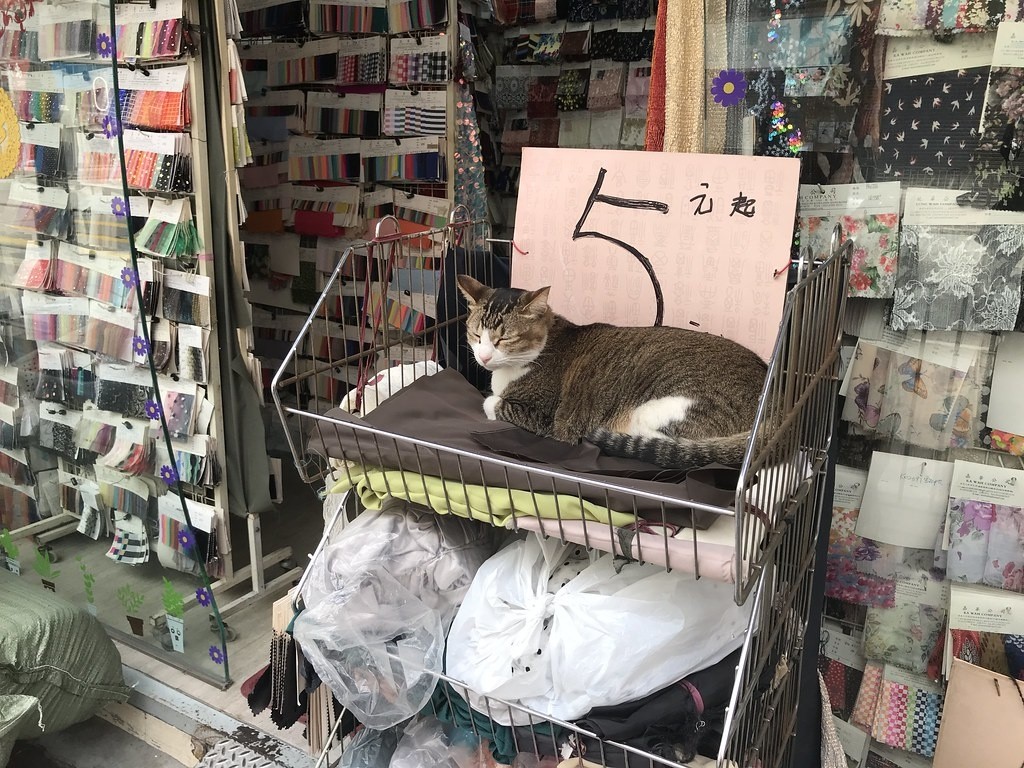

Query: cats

[456,274,791,466]
